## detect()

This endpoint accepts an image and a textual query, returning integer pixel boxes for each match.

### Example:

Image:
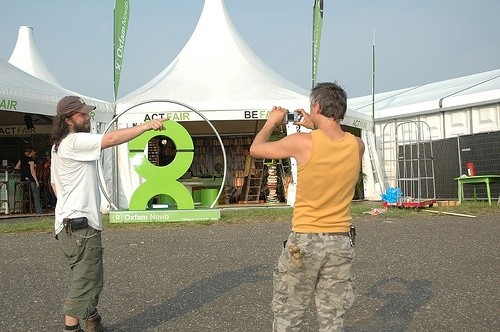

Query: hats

[57,96,96,115]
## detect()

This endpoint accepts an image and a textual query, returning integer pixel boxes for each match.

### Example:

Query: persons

[47,94,172,332]
[249,80,365,332]
[15,147,57,213]
[239,147,267,202]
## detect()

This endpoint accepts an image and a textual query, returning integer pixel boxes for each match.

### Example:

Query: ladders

[362,130,380,195]
[245,156,264,203]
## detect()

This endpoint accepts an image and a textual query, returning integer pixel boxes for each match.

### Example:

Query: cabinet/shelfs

[188,136,268,202]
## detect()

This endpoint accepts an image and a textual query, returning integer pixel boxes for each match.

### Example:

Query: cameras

[285,112,299,122]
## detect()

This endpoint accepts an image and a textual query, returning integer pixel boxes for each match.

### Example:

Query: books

[266,165,280,204]
[148,136,254,188]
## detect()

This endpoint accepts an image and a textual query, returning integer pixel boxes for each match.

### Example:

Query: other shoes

[63,325,84,332]
[86,314,104,332]
[37,209,46,214]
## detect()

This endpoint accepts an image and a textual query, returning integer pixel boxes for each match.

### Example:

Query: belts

[293,231,349,236]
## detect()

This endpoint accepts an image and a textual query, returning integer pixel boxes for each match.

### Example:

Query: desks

[157,176,228,208]
[453,175,500,206]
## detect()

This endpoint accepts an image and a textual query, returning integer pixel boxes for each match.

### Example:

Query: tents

[344,67,499,201]
[8,25,62,89]
[0,59,130,219]
[110,0,390,209]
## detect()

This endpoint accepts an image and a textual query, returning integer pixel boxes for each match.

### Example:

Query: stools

[0,180,11,215]
[39,182,48,214]
[13,181,33,215]
